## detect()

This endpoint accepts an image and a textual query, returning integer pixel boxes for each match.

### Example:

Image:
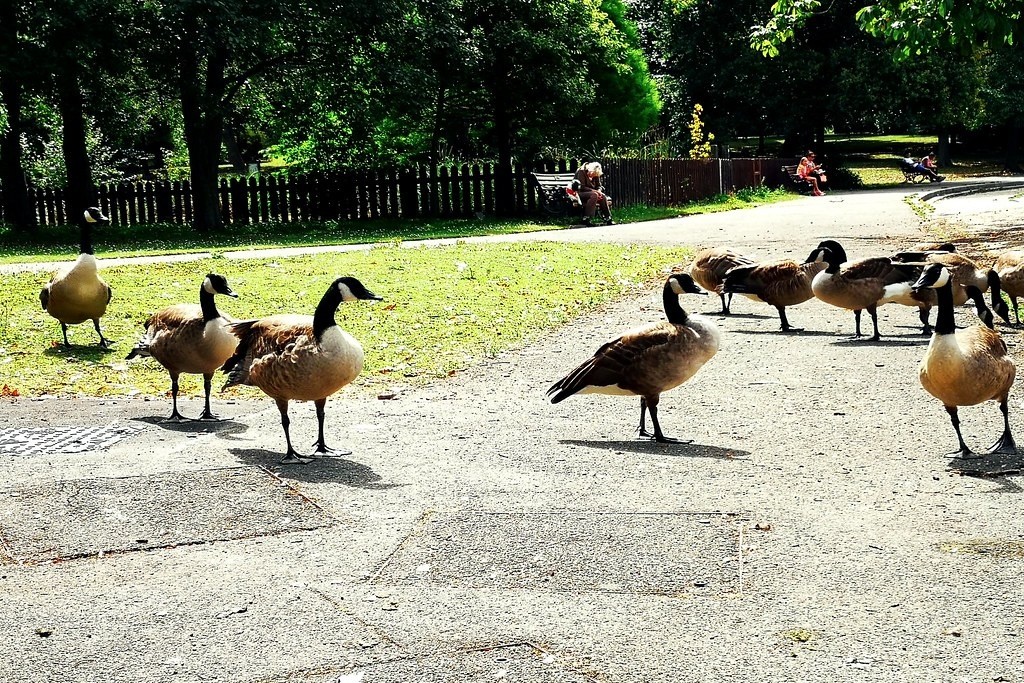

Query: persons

[807,152,822,190]
[574,162,616,227]
[797,156,826,196]
[903,151,946,182]
[923,152,937,182]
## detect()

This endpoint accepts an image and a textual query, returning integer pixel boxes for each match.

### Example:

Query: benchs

[781,162,827,195]
[530,172,613,225]
[896,159,938,184]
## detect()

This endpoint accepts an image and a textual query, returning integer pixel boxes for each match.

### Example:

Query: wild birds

[221,277,386,465]
[39,206,117,350]
[688,239,1024,342]
[909,258,1024,458]
[543,274,718,442]
[125,272,242,423]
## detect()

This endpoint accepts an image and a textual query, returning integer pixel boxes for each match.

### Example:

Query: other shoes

[586,218,600,227]
[936,177,946,182]
[811,192,819,196]
[604,219,616,225]
[817,190,824,196]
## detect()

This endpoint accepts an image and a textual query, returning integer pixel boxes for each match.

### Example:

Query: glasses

[810,156,815,158]
